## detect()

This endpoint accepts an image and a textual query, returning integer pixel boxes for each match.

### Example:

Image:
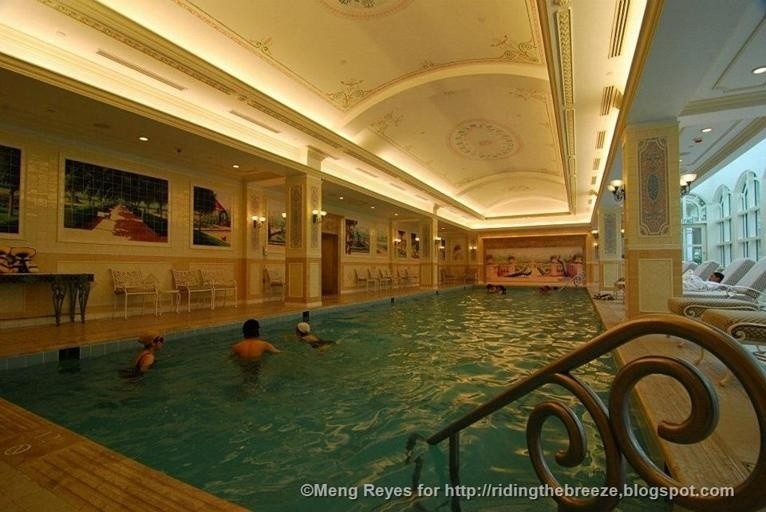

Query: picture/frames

[0,143,421,262]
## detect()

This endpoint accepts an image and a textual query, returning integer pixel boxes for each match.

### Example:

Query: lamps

[607,179,626,202]
[680,173,698,196]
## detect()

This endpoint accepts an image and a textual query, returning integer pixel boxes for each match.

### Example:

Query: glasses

[156,337,163,342]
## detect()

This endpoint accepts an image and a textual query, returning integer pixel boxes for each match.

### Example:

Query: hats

[297,322,310,333]
[138,330,159,343]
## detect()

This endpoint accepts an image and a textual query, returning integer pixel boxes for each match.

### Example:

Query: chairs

[613,259,766,388]
[265,262,287,302]
[354,264,420,292]
[109,269,237,321]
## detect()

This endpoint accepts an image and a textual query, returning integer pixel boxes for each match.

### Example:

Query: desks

[0,271,93,327]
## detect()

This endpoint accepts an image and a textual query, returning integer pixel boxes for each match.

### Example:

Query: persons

[134,331,164,374]
[539,285,558,295]
[295,322,334,351]
[708,272,723,281]
[229,319,280,360]
[487,283,507,295]
